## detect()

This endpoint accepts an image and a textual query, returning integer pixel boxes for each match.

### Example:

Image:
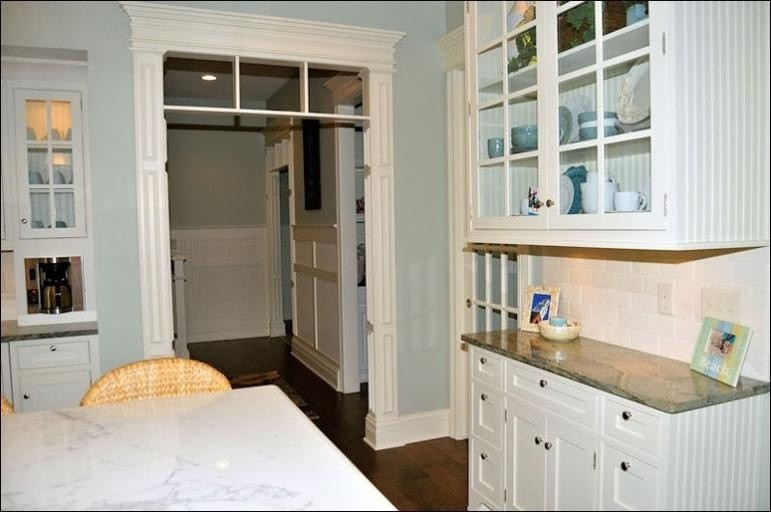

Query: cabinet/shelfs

[466,343,771,512]
[464,1,771,251]
[1,334,100,413]
[1,78,96,328]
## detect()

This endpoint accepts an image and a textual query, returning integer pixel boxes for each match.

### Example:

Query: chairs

[79,357,231,407]
[1,396,15,415]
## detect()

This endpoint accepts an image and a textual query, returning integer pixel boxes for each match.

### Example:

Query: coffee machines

[38,257,74,316]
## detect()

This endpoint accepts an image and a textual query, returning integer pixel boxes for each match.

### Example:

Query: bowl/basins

[486,94,619,159]
[537,318,582,344]
[27,125,75,228]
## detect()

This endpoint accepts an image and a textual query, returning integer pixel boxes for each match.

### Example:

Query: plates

[560,165,587,214]
[615,54,650,125]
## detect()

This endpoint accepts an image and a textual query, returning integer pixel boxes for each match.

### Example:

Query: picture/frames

[521,285,560,333]
[689,317,751,387]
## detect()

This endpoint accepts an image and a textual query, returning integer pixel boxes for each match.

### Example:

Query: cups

[549,317,568,328]
[579,170,650,216]
[625,2,647,27]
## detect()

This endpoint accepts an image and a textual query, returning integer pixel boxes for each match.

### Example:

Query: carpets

[229,370,319,421]
